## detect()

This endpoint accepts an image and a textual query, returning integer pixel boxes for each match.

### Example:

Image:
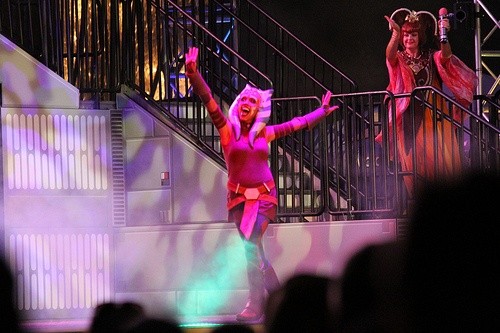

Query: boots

[235,264,279,321]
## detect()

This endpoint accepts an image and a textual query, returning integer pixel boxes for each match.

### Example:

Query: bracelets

[392,31,398,39]
[322,104,329,114]
[184,69,198,78]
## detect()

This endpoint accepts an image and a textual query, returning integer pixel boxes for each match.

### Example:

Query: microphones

[438,8,448,44]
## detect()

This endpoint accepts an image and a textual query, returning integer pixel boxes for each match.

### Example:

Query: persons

[375,8,476,216]
[0,253,22,333]
[185,47,339,324]
[86,167,500,333]
